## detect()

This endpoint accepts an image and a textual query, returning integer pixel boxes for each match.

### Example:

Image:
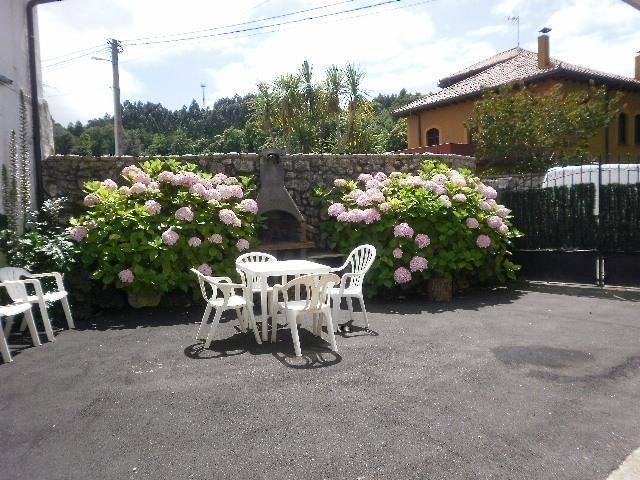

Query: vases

[427,276,452,301]
[128,289,162,309]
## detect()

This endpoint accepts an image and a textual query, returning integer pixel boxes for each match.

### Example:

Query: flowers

[314,154,526,290]
[73,153,260,300]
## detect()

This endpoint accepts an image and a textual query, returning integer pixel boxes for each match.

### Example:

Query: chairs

[187,241,379,361]
[0,263,76,365]
